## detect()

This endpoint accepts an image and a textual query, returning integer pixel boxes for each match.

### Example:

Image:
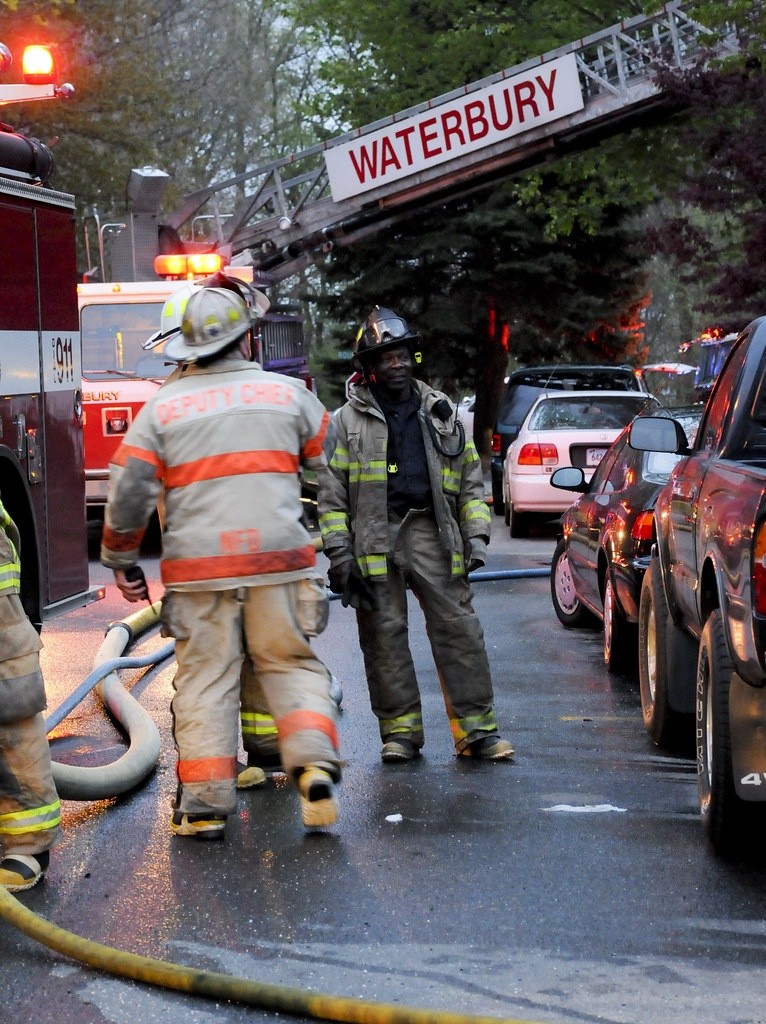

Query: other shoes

[168,811,227,838]
[461,735,515,759]
[236,759,267,789]
[247,751,285,772]
[380,739,418,759]
[0,848,49,892]
[298,765,338,828]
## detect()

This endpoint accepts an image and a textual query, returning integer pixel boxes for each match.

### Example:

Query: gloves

[327,557,381,613]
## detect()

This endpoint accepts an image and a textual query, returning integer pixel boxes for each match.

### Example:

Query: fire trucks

[0,1,754,637]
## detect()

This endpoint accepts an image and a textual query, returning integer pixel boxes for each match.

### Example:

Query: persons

[101,273,344,835]
[0,500,59,894]
[318,302,517,763]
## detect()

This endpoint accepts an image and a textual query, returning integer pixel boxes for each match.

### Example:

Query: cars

[489,314,766,848]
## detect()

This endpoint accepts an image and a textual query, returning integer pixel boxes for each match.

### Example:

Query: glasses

[360,317,408,348]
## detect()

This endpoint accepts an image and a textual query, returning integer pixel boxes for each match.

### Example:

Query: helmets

[350,308,426,360]
[141,278,203,351]
[164,273,270,362]
